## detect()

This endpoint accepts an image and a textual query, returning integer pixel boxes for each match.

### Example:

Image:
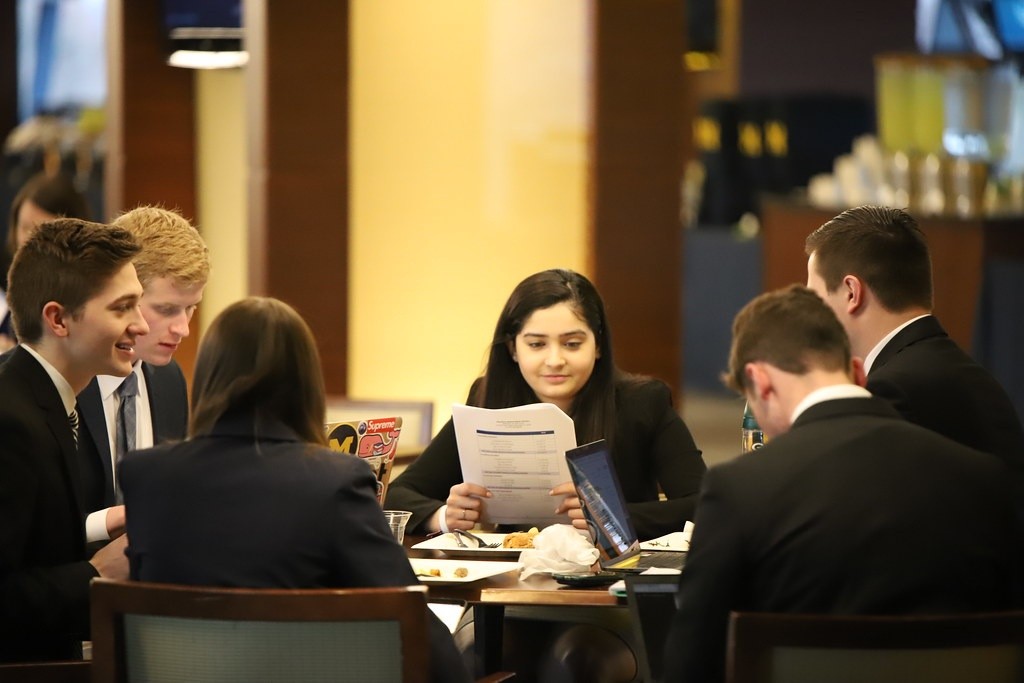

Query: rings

[463,509,466,521]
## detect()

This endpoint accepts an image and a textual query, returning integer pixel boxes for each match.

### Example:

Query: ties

[115,371,136,508]
[69,410,79,454]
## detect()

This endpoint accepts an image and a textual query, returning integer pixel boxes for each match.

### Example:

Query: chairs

[323,395,435,488]
[89,576,515,683]
[725,610,1024,683]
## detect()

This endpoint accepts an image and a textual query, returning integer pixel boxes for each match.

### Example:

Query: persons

[384,270,711,682]
[113,294,472,683]
[0,206,216,683]
[664,208,1019,683]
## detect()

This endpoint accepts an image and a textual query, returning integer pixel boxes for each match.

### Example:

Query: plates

[410,533,533,556]
[640,532,692,551]
[408,558,525,584]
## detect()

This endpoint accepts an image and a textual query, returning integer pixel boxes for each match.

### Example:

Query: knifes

[452,532,468,548]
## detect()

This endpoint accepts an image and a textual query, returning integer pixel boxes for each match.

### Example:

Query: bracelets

[439,505,450,534]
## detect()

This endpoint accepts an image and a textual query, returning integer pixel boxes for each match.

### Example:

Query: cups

[383,510,413,545]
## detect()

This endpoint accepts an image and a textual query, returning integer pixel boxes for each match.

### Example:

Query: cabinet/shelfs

[759,195,1024,407]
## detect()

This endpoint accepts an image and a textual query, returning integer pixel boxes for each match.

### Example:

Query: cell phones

[552,571,628,582]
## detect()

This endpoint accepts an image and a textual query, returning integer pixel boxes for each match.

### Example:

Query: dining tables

[396,534,620,683]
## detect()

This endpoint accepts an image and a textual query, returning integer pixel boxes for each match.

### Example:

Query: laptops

[324,417,403,509]
[565,439,688,575]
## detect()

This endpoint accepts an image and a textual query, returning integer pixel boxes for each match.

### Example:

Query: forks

[454,529,502,548]
